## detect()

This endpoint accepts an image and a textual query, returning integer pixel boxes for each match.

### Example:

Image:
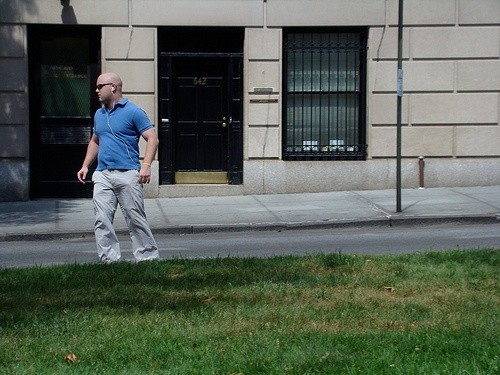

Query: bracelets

[142,163,151,167]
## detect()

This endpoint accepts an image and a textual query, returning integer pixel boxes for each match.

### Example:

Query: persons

[77,73,159,264]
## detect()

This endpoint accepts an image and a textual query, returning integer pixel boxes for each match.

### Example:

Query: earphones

[112,87,114,90]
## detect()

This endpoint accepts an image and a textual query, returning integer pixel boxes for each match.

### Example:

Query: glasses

[96,83,112,90]
